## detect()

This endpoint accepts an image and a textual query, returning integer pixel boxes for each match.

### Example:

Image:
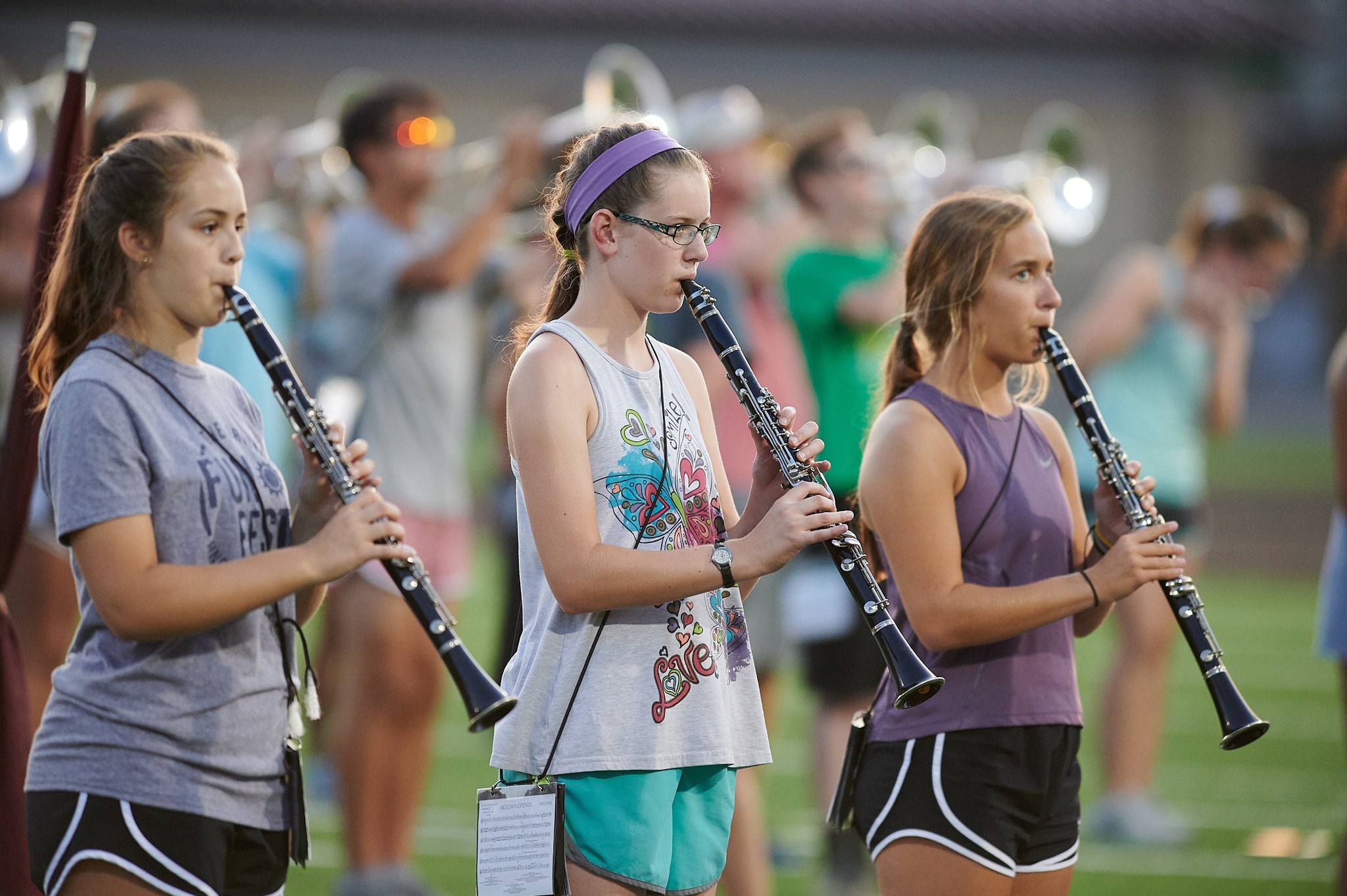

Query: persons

[1065,185,1308,849]
[22,134,416,895]
[856,195,1186,896]
[490,124,855,896]
[1,76,1027,894]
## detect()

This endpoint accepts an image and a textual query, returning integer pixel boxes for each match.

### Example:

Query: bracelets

[1080,571,1099,608]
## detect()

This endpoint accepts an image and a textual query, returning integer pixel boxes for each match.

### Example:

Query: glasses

[580,206,720,246]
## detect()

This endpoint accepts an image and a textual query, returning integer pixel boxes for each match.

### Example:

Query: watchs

[710,542,736,588]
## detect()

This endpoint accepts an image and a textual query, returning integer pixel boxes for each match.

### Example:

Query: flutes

[1040,322,1269,751]
[679,270,945,707]
[220,282,517,737]
